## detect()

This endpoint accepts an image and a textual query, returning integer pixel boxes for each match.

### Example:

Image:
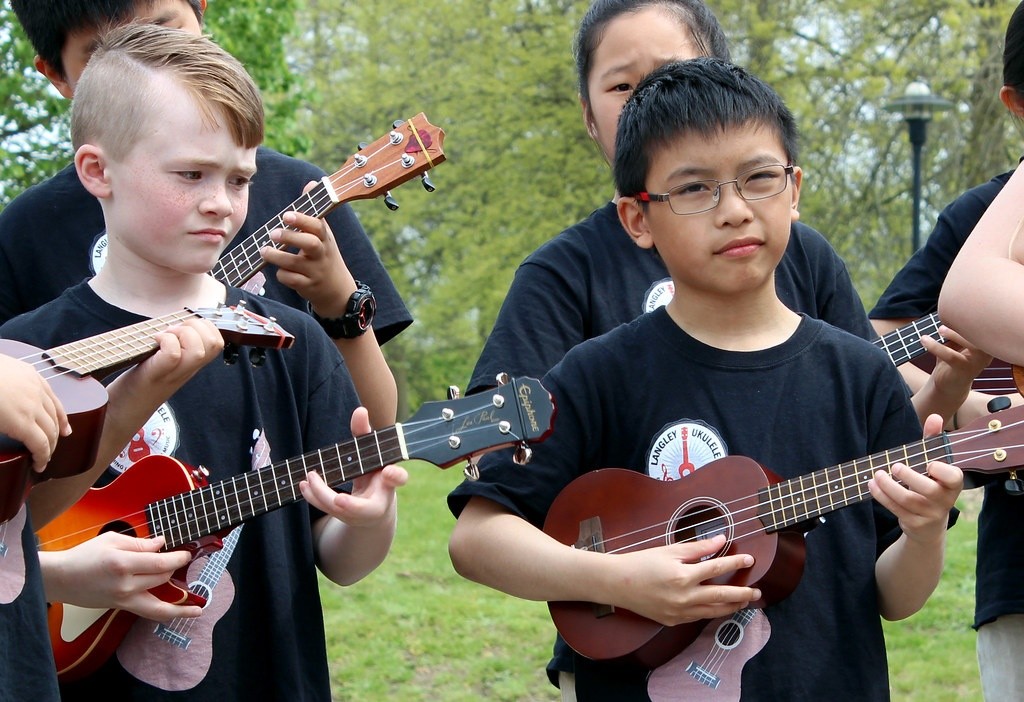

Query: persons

[864,0,1024,702]
[463,0,996,439]
[0,0,419,702]
[448,58,963,702]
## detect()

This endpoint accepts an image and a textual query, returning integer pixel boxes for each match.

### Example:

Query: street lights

[882,80,965,259]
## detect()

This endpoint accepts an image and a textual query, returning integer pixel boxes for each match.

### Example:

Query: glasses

[634,163,795,216]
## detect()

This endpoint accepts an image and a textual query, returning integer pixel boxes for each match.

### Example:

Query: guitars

[34,368,557,678]
[547,395,1024,661]
[206,110,447,289]
[873,312,948,366]
[966,359,1024,403]
[0,300,299,522]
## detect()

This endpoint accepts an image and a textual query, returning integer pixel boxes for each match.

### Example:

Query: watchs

[306,281,375,339]
